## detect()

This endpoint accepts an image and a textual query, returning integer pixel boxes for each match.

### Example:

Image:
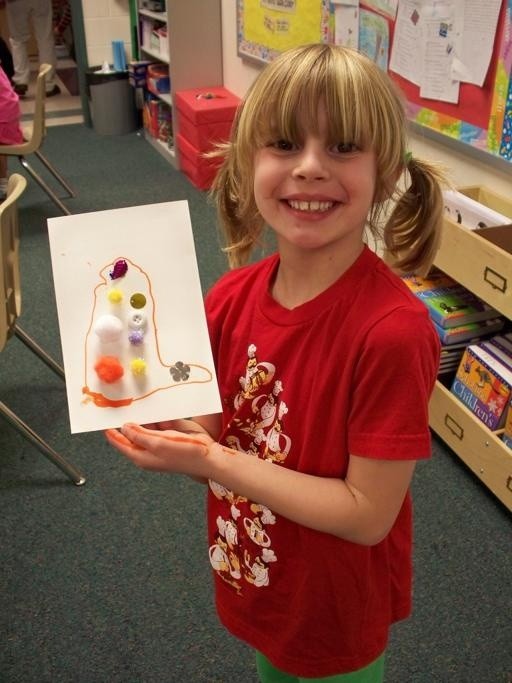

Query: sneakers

[45,84,61,97]
[14,84,28,95]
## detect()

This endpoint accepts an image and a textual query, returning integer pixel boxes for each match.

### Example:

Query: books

[392,266,512,455]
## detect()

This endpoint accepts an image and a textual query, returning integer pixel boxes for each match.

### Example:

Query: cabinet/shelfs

[136,0,223,171]
[381,184,512,514]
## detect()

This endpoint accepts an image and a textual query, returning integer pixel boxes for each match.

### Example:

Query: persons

[93,43,440,682]
[0,65,24,202]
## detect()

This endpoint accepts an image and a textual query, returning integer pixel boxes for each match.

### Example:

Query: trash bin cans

[86,66,136,135]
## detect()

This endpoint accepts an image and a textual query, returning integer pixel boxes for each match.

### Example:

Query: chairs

[0,63,76,216]
[0,172,87,486]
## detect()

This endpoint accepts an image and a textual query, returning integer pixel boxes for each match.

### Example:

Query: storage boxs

[176,134,226,191]
[175,86,245,153]
